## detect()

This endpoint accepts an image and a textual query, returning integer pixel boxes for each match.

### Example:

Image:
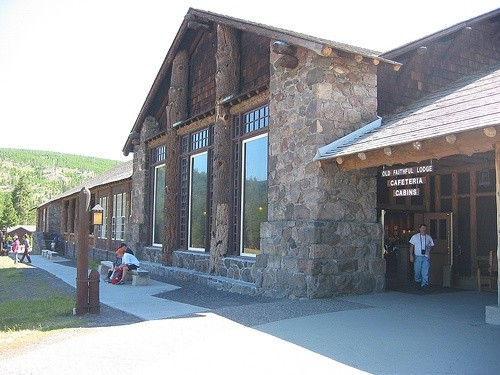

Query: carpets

[391,280,464,296]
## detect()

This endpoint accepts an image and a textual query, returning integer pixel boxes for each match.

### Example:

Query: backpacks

[112,268,123,284]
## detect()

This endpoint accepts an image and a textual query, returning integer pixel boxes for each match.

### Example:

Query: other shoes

[415,282,420,288]
[422,287,428,292]
[116,280,125,285]
[103,276,110,280]
[105,277,112,282]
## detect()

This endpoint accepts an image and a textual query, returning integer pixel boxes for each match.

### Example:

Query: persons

[103,243,134,282]
[116,248,141,285]
[12,236,20,264]
[408,224,435,289]
[20,234,32,263]
[3,239,11,256]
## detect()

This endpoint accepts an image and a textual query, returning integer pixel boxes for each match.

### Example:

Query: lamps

[92,204,104,226]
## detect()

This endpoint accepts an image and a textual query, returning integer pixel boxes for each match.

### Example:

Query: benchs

[42,249,58,259]
[99,261,151,288]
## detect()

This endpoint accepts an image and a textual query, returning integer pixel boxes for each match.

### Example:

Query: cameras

[421,250,425,254]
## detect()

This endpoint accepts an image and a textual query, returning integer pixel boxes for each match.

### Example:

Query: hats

[119,243,126,248]
[116,249,124,253]
